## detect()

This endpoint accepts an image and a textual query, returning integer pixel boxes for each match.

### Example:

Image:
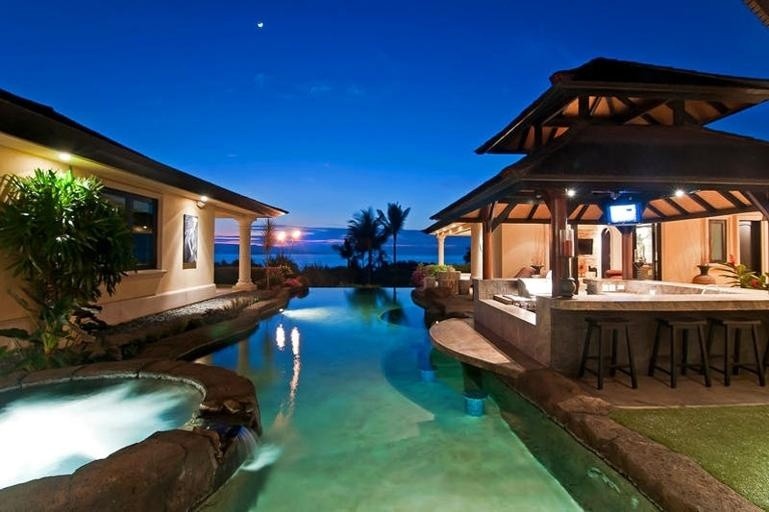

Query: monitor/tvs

[607,201,641,223]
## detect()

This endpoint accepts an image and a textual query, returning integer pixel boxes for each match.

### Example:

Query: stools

[576,316,766,390]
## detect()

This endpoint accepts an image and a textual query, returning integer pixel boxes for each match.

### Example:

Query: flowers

[712,253,769,290]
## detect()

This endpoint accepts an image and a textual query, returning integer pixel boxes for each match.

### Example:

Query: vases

[692,265,716,284]
